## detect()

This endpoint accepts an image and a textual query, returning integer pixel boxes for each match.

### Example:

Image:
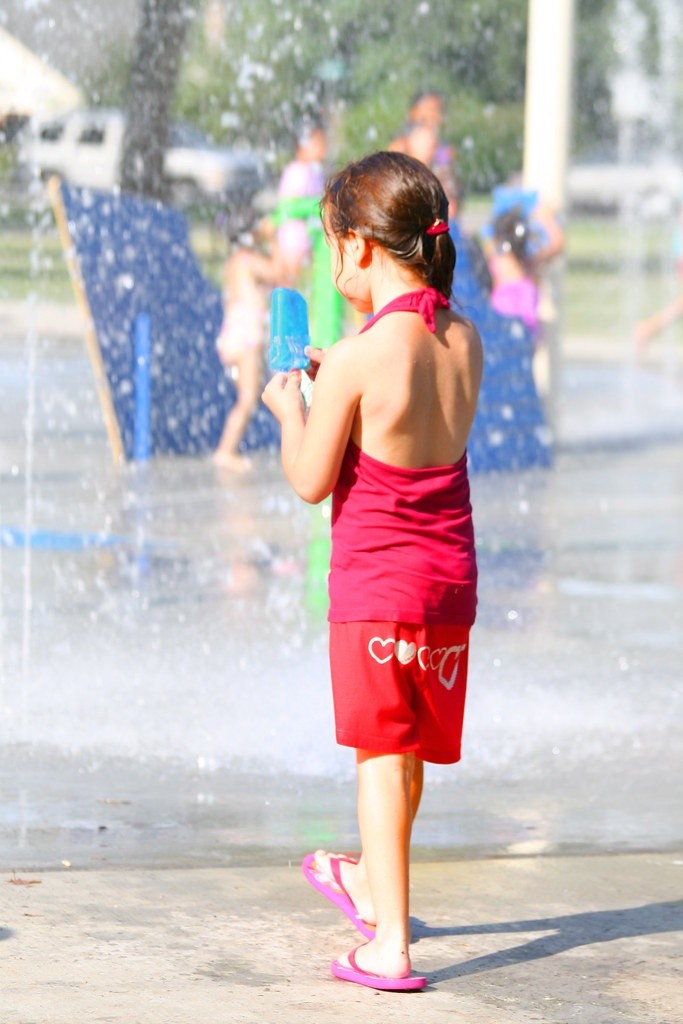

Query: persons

[261,151,485,990]
[212,89,683,472]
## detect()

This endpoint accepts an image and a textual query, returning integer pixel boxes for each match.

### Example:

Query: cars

[16,101,272,214]
[563,134,683,222]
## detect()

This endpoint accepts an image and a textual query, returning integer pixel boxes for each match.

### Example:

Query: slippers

[303,853,377,939]
[331,941,427,989]
